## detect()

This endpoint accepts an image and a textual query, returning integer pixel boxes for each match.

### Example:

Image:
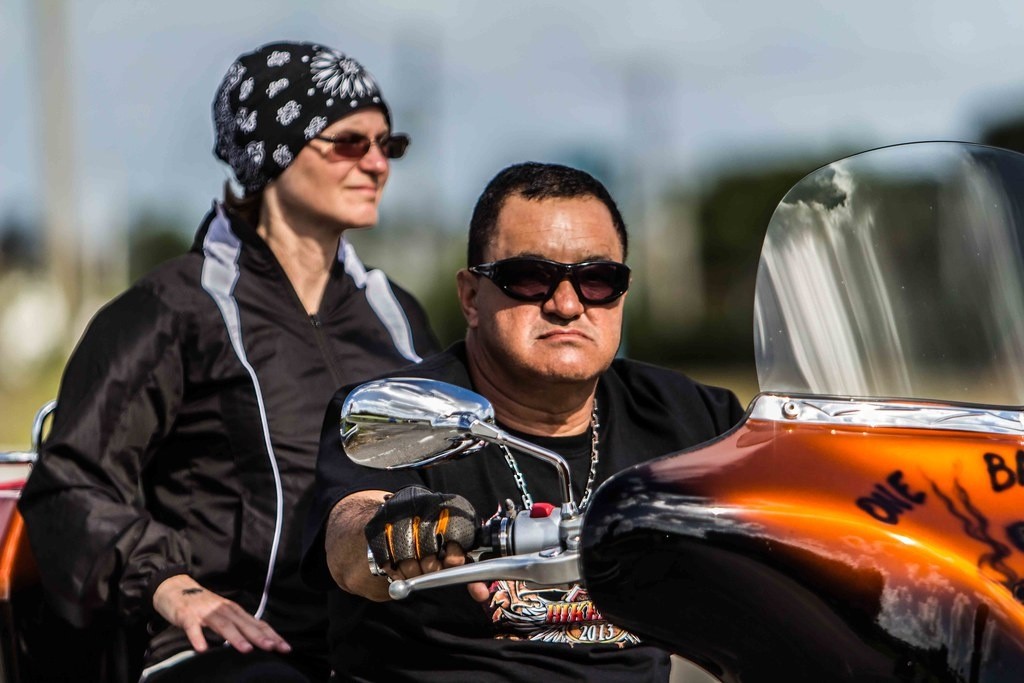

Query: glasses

[315,130,410,159]
[468,254,634,307]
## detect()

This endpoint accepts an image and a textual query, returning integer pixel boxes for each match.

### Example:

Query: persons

[17,40,441,683]
[314,163,750,683]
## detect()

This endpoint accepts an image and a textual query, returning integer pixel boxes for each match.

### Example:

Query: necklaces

[499,401,600,509]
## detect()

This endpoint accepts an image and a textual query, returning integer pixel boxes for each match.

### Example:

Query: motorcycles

[0,141,1024,683]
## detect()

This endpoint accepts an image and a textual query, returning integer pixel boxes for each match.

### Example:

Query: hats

[211,40,391,194]
